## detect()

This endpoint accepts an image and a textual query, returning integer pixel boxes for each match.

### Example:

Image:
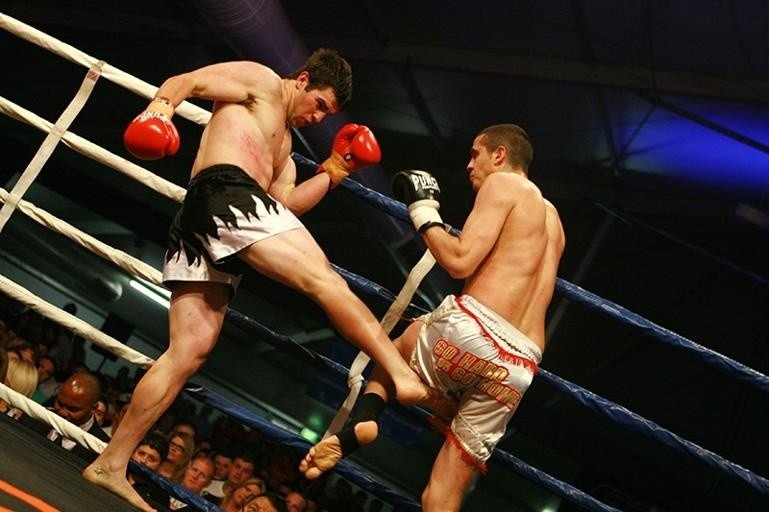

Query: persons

[296,122,568,512]
[132,392,385,511]
[1,301,147,463]
[79,49,459,512]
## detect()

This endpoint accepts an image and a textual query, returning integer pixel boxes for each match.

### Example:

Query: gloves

[314,122,382,190]
[390,168,447,239]
[122,96,181,162]
